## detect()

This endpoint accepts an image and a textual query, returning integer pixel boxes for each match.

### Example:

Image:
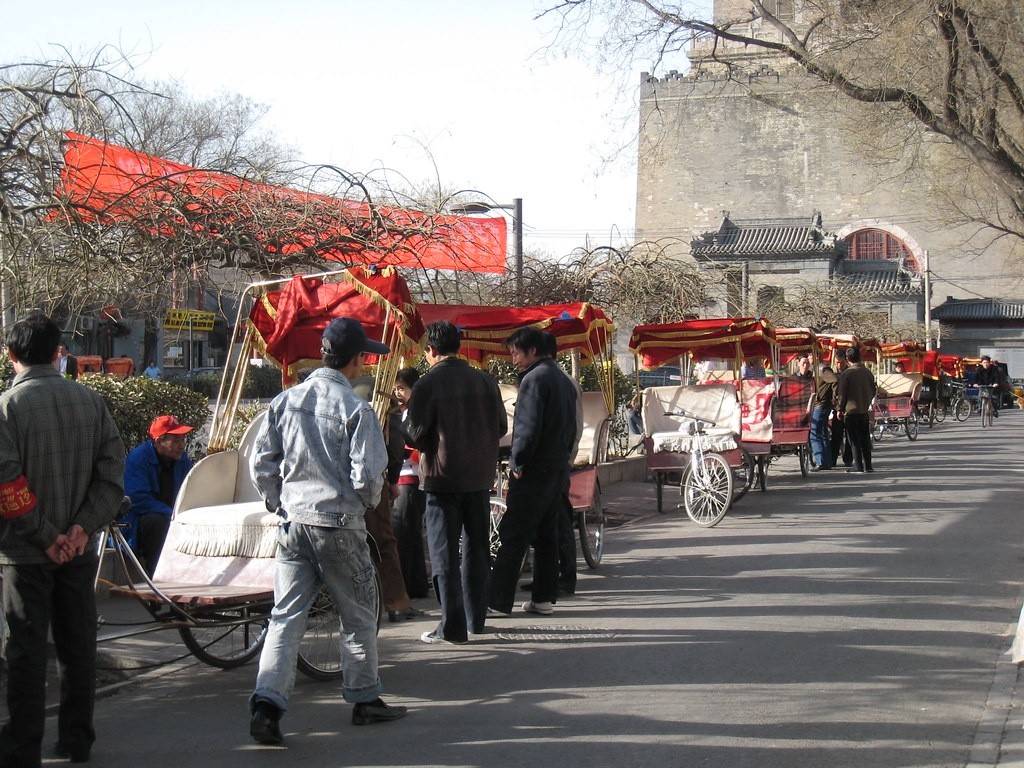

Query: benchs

[696,377,781,440]
[642,383,741,453]
[499,383,519,449]
[756,377,815,432]
[872,371,923,404]
[175,408,294,555]
[574,391,611,463]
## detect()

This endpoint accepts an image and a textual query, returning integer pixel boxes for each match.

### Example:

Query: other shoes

[520,582,577,600]
[866,467,873,472]
[388,606,424,622]
[846,468,864,472]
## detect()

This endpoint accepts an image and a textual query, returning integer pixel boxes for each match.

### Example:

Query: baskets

[979,387,994,397]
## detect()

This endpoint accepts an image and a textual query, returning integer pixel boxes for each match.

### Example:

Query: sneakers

[486,606,510,618]
[522,600,553,615]
[421,627,468,646]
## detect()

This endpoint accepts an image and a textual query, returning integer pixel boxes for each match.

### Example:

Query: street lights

[701,261,749,318]
[449,197,523,307]
[189,316,207,378]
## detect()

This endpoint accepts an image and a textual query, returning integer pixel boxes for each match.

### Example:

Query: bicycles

[976,383,998,428]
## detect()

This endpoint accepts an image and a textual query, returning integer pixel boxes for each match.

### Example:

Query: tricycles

[629,317,1008,529]
[44,261,617,717]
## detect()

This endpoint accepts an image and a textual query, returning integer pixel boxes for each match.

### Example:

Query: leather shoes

[249,702,284,745]
[810,464,831,472]
[54,742,89,763]
[352,698,407,726]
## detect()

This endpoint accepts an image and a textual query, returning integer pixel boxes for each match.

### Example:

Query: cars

[186,367,222,377]
[627,366,698,396]
[1003,374,1015,408]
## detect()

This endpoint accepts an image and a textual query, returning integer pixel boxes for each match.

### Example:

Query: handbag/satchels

[843,429,853,463]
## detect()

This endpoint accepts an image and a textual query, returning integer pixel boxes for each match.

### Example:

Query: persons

[890,362,906,374]
[58,343,78,382]
[143,360,162,378]
[0,312,126,768]
[345,320,584,646]
[968,356,1005,418]
[692,359,718,385]
[739,356,766,380]
[247,317,407,745]
[107,415,194,612]
[789,345,876,474]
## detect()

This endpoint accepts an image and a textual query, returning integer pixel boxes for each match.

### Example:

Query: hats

[320,317,390,357]
[149,415,193,440]
